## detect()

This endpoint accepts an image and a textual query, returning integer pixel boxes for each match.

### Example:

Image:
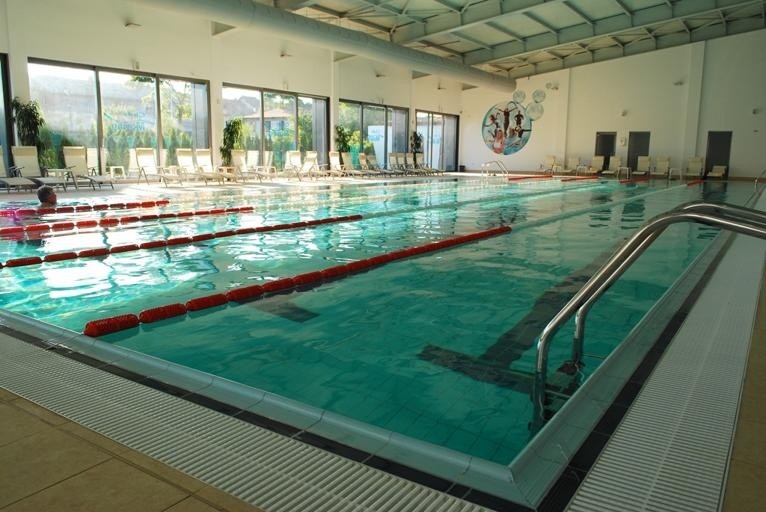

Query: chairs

[416,154,446,176]
[86,147,108,176]
[388,153,407,177]
[0,145,37,194]
[11,146,67,194]
[542,156,728,181]
[397,153,419,176]
[231,148,261,184]
[133,147,182,186]
[176,148,208,184]
[405,153,428,176]
[195,149,239,184]
[258,150,280,180]
[283,149,394,179]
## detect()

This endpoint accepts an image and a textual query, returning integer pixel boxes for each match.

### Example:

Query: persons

[485,107,531,154]
[37,187,56,208]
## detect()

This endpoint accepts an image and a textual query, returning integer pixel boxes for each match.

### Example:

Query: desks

[104,165,128,181]
[44,169,80,191]
[62,146,116,190]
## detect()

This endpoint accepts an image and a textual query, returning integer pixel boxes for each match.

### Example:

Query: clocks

[618,136,625,146]
